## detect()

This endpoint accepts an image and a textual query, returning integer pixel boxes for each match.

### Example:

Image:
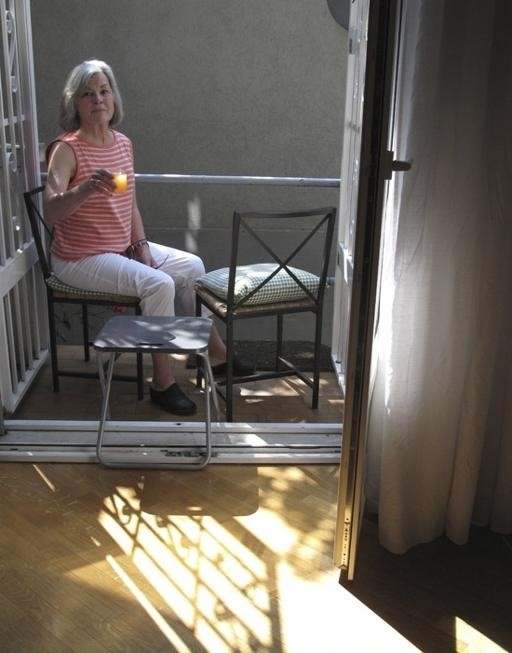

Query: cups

[110,169,127,195]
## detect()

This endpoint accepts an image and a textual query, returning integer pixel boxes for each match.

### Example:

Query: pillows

[196,262,328,307]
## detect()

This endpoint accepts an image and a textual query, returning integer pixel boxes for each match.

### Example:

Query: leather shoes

[211,362,257,376]
[148,380,198,416]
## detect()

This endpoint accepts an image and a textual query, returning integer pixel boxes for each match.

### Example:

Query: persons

[43,60,256,415]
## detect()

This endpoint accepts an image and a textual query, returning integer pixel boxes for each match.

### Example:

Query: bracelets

[124,239,147,254]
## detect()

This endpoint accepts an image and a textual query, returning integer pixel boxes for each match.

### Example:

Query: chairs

[23,184,143,399]
[195,208,336,422]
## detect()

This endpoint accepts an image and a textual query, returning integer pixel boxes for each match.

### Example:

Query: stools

[92,314,220,470]
